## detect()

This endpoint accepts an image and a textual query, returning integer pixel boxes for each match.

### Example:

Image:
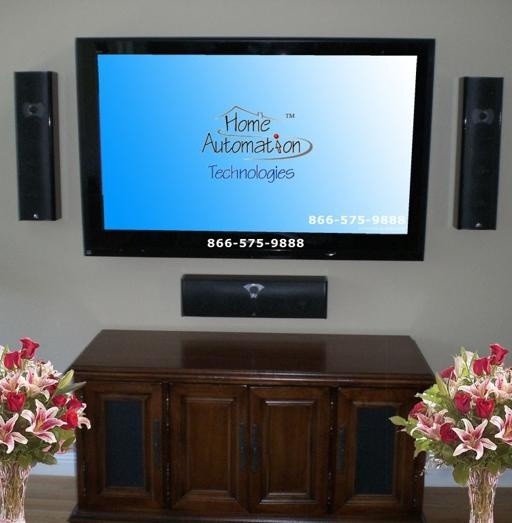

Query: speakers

[453,77,503,231]
[15,71,62,221]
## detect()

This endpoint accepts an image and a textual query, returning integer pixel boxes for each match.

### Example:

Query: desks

[63,330,436,523]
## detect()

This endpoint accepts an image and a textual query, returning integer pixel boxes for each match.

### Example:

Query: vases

[467,467,498,523]
[0,460,32,523]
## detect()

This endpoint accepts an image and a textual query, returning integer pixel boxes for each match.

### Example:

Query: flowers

[0,339,91,523]
[389,343,512,523]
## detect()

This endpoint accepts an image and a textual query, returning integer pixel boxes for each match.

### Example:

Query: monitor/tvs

[75,36,435,262]
